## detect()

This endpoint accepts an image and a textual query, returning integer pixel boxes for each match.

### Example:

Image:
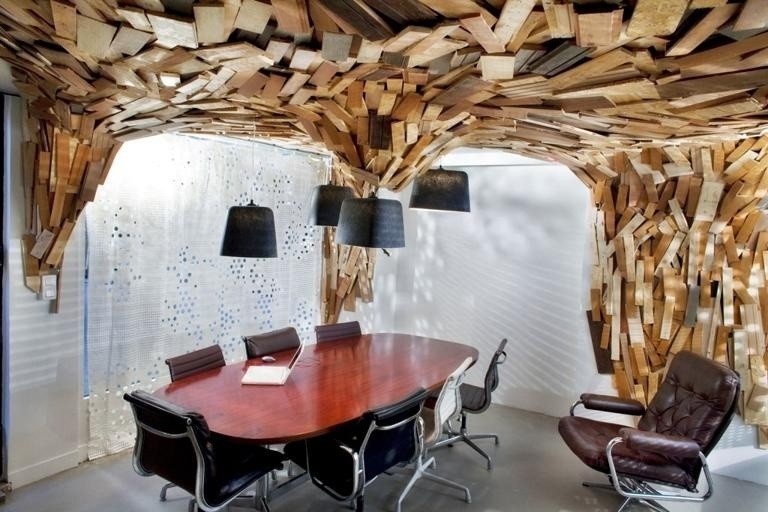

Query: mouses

[261,355,275,363]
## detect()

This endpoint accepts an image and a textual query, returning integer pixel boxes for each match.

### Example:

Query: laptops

[239,336,309,387]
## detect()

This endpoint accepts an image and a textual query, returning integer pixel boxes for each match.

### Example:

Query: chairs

[125,390,286,512]
[559,350,740,512]
[396,353,476,512]
[167,342,277,481]
[282,384,428,512]
[422,338,509,469]
[243,325,304,359]
[316,323,362,344]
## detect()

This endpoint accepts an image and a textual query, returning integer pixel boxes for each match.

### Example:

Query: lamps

[222,117,470,258]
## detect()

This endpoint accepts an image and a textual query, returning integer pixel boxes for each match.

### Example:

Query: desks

[152,333,479,511]
